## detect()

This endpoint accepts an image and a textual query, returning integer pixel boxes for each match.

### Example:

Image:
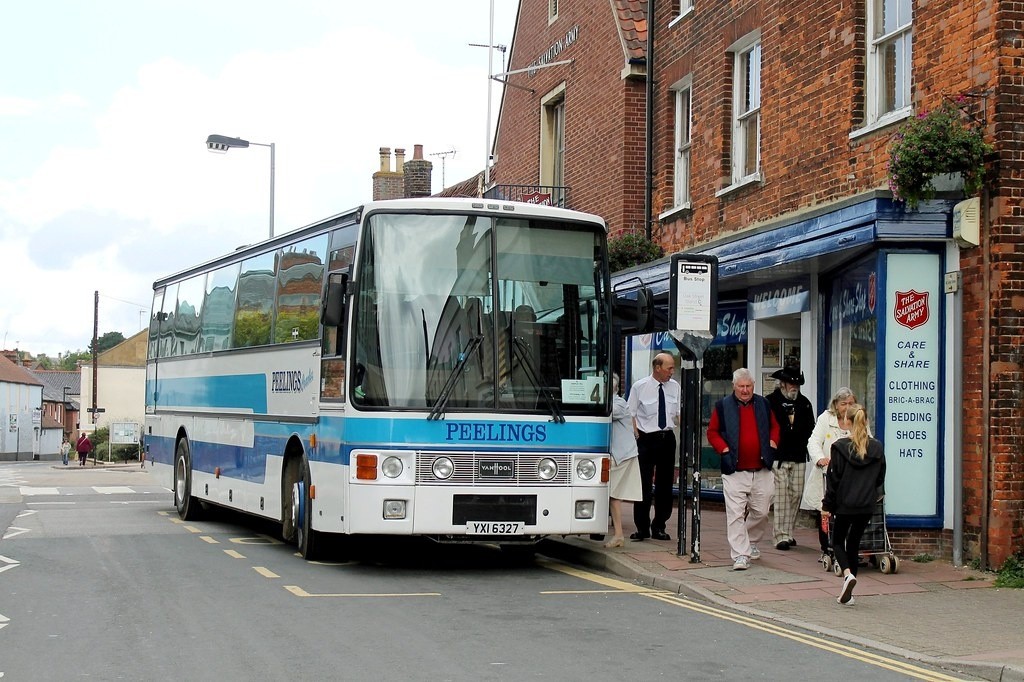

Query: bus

[143,197,655,562]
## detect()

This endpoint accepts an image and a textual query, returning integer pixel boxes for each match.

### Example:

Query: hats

[771,362,805,385]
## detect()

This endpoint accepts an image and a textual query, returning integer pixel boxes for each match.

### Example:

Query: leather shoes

[630,531,651,539]
[651,529,671,540]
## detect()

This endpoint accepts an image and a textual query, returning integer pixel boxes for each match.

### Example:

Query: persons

[820,404,886,606]
[627,352,681,540]
[75,433,93,466]
[60,438,71,465]
[603,373,643,548]
[798,386,872,562]
[764,358,816,550]
[708,368,780,570]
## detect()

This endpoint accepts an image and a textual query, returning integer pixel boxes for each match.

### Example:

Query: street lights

[63,386,71,439]
[206,134,274,237]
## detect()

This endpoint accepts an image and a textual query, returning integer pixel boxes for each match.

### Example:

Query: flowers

[605,225,668,276]
[885,91,995,213]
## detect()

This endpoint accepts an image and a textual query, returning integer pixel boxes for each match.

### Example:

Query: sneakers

[836,594,856,606]
[749,544,761,560]
[838,573,857,604]
[732,556,751,570]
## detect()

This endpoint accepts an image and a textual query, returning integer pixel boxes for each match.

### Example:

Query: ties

[658,383,666,430]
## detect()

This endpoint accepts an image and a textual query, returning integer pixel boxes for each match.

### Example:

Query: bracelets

[635,434,639,439]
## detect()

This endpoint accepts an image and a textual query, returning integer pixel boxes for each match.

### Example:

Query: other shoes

[788,538,797,546]
[603,535,625,549]
[776,541,790,550]
[80,461,82,466]
[83,465,85,466]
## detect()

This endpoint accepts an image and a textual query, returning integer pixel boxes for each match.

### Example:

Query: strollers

[821,463,899,577]
